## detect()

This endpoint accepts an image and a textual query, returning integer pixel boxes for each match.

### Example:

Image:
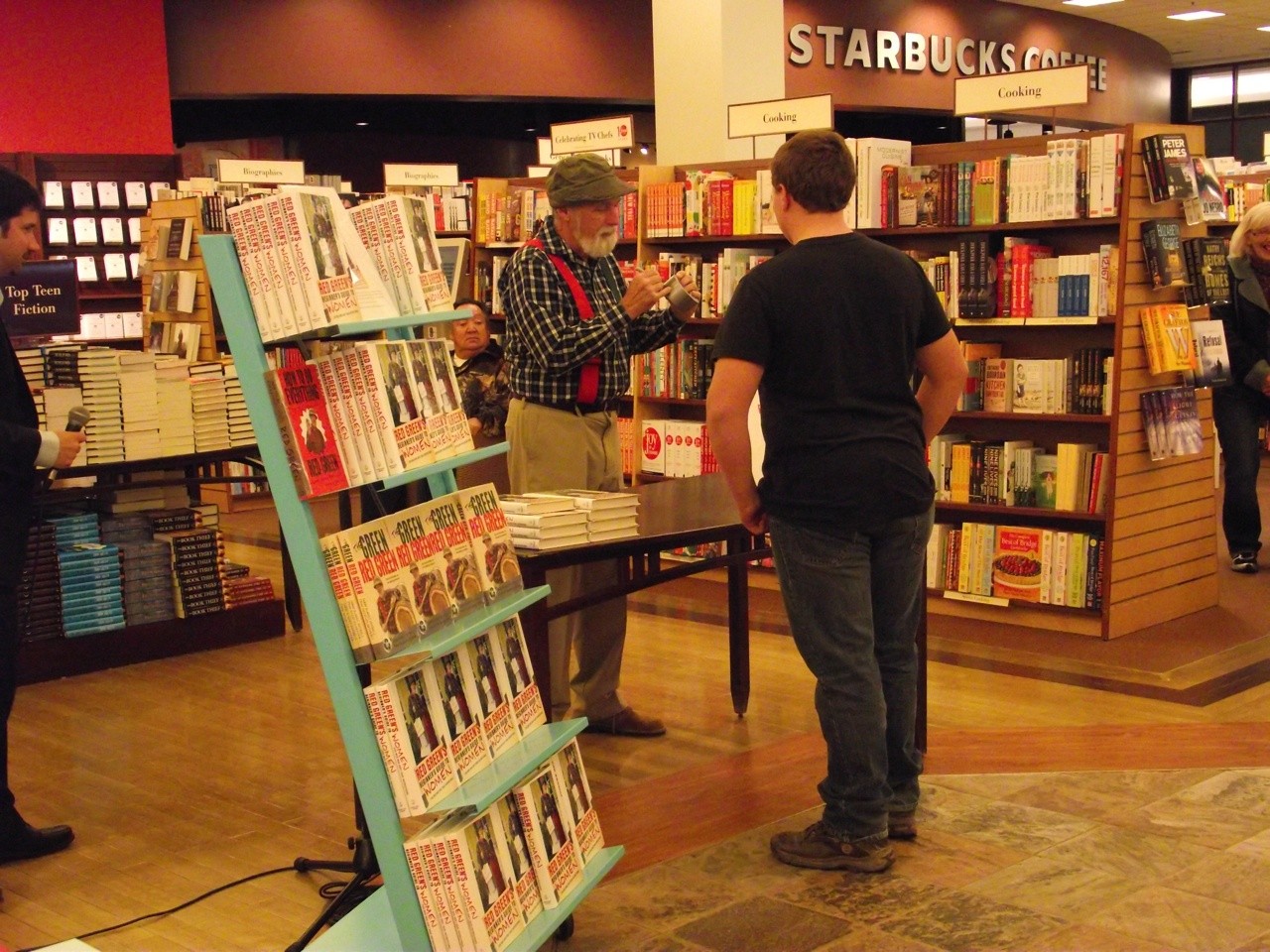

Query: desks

[511,473,771,726]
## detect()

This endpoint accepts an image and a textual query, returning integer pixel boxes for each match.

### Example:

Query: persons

[0,165,86,864]
[496,153,701,736]
[1210,202,1270,573]
[705,130,968,873]
[449,299,513,449]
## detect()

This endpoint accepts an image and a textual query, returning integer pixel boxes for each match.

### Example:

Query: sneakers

[891,812,916,837]
[770,816,897,872]
[1231,549,1258,572]
[584,707,664,734]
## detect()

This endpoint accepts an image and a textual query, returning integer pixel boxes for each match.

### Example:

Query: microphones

[44,407,91,487]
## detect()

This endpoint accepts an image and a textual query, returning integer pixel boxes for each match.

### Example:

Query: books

[1139,217,1231,461]
[13,178,258,470]
[499,490,640,549]
[403,738,604,952]
[19,456,275,643]
[904,340,1114,415]
[925,433,1110,514]
[319,483,546,818]
[926,522,1106,609]
[475,170,783,318]
[263,340,475,500]
[618,334,775,569]
[1140,133,1270,225]
[842,134,1126,319]
[225,175,476,343]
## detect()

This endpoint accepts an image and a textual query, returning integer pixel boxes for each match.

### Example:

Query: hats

[546,153,638,207]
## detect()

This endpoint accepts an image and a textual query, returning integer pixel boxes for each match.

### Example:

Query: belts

[513,388,619,415]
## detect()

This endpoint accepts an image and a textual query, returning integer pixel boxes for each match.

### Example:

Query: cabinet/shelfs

[196,232,628,952]
[17,445,303,690]
[469,121,1222,644]
[136,197,474,513]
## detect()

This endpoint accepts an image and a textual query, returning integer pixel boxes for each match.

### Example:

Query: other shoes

[0,822,76,863]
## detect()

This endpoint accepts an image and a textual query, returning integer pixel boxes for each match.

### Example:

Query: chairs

[407,434,511,504]
